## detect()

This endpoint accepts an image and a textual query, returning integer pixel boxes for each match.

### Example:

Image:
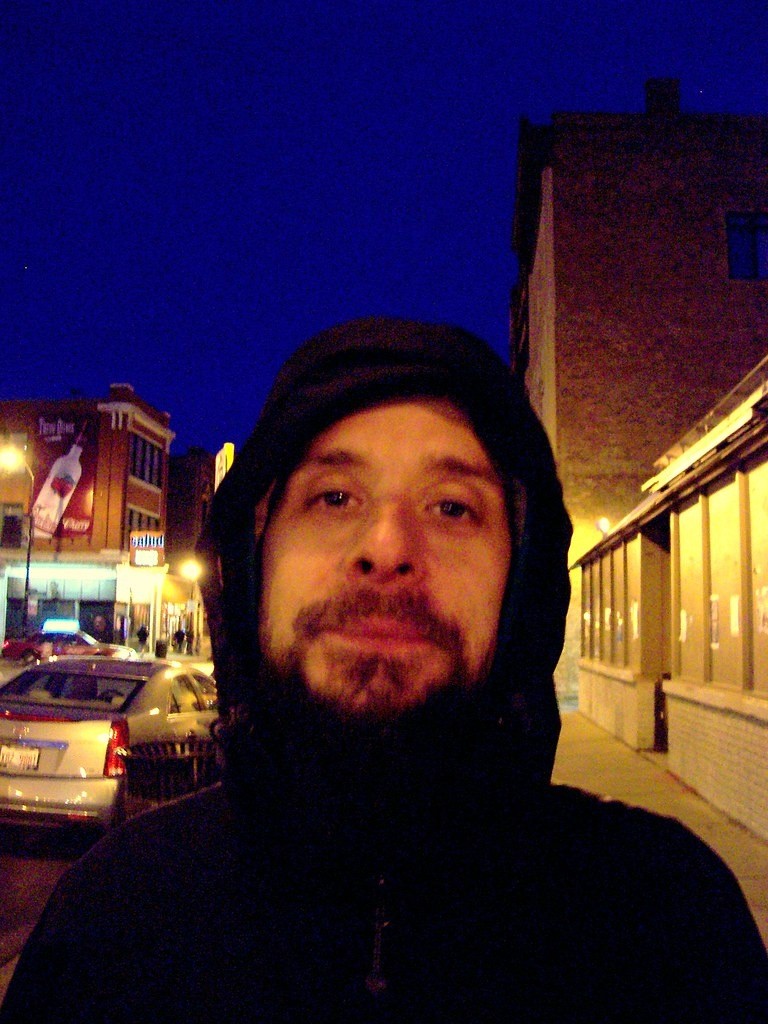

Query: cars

[0,658,221,829]
[3,619,140,663]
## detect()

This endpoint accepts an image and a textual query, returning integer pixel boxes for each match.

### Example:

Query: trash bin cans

[111,738,218,824]
[155,640,167,658]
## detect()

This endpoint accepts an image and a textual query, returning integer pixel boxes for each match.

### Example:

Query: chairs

[29,686,52,699]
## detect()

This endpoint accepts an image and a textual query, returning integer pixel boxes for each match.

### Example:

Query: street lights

[0,445,31,623]
[179,560,200,656]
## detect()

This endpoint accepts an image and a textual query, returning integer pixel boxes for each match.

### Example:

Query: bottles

[30,417,94,539]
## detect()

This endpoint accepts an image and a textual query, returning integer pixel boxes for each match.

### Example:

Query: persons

[137,626,150,653]
[97,620,115,644]
[173,626,194,657]
[0,319,768,1024]
